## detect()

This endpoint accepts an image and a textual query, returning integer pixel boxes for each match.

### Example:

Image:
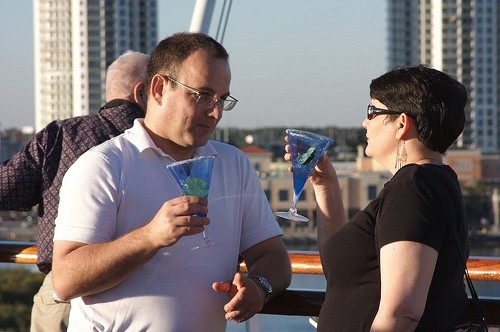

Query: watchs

[247,276,272,302]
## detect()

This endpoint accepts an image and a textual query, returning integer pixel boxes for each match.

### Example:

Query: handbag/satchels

[415,324,488,332]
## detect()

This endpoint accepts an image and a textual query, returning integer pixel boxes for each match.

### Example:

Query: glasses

[163,75,238,111]
[367,105,401,120]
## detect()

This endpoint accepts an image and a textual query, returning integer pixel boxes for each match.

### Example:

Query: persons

[284,66,471,332]
[52,33,291,332]
[0,50,150,332]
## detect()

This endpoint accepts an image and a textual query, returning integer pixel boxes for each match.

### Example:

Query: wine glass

[274,129,333,222]
[165,156,221,250]
